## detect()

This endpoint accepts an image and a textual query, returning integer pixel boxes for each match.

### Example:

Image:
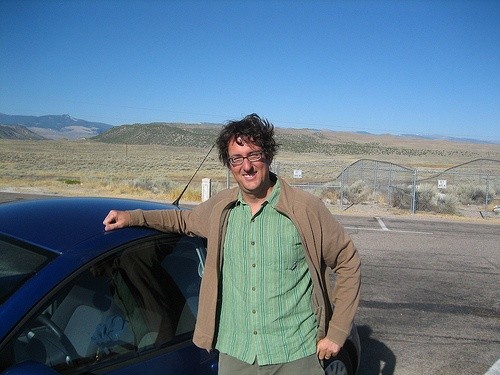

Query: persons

[86,290,150,358]
[102,113,363,375]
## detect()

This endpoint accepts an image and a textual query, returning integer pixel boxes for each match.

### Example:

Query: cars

[0,196,362,375]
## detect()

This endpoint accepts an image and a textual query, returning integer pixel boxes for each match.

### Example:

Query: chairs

[8,249,173,372]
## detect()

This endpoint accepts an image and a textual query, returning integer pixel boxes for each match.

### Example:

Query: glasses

[228,150,267,163]
